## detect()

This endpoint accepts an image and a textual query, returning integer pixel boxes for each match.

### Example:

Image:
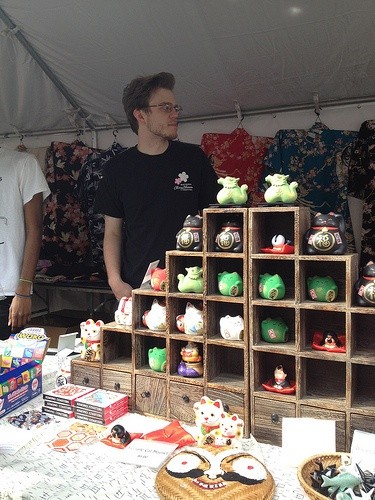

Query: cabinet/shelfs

[72,206,375,453]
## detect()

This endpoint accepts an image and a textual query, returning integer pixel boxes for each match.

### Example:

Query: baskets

[155,444,275,500]
[296,451,375,500]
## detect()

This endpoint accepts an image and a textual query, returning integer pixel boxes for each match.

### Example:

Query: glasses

[140,104,183,113]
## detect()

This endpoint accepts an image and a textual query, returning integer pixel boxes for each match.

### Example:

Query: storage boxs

[23,325,68,347]
[0,328,51,419]
[56,332,82,373]
[283,417,347,457]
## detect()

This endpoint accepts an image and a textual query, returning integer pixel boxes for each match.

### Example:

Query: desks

[31,279,117,322]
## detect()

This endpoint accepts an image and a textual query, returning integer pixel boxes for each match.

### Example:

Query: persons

[94,71,222,304]
[322,332,339,348]
[272,365,293,390]
[0,150,53,342]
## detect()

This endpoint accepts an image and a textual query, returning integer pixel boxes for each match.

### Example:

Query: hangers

[113,130,119,142]
[19,136,24,146]
[76,130,81,140]
[238,115,245,129]
[314,106,322,122]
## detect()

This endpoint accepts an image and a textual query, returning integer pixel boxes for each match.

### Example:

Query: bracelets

[19,279,32,283]
[14,292,33,299]
